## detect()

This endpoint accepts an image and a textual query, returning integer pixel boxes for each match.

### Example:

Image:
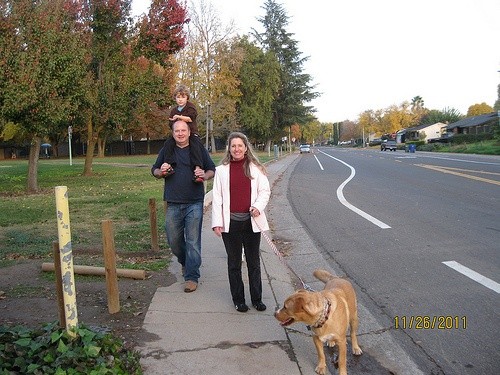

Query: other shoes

[193,174,204,183]
[183,280,198,293]
[252,301,266,311]
[161,167,175,177]
[181,266,185,276]
[234,302,251,313]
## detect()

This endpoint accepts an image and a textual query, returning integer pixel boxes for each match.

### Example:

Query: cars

[300,144,311,154]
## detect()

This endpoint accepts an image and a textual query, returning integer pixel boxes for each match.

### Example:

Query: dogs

[274,269,363,375]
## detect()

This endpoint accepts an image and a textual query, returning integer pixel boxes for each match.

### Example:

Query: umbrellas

[41,144,52,159]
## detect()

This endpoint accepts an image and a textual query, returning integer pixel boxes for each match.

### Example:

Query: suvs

[380,139,397,152]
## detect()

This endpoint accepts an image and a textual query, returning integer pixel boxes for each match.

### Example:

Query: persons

[151,120,215,293]
[212,132,271,311]
[163,84,203,184]
